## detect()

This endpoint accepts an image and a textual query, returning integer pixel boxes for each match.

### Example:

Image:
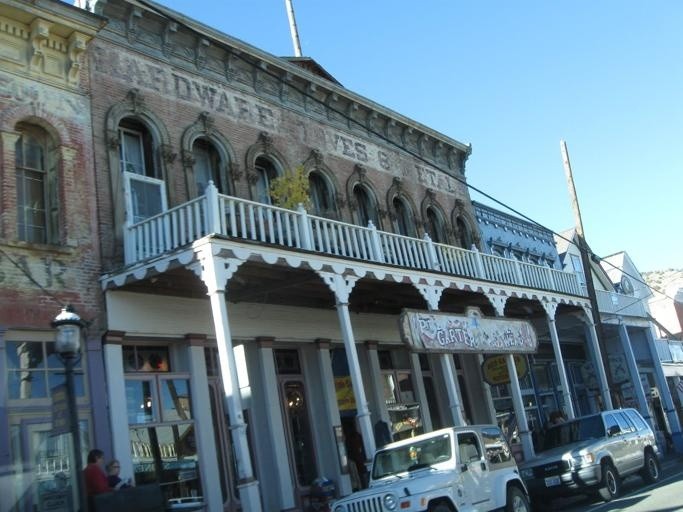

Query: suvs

[518,407,663,503]
[329,423,533,512]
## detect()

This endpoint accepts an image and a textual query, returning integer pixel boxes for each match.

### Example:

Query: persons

[544,410,563,431]
[406,446,421,468]
[83,448,115,496]
[105,458,135,488]
